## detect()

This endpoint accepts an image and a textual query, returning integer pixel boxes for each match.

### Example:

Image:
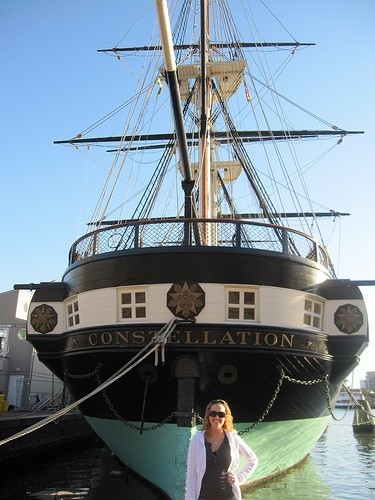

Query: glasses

[208,411,225,418]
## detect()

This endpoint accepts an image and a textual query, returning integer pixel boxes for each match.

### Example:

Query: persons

[185,400,258,500]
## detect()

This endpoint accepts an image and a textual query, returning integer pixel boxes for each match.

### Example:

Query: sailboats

[14,0,369,500]
